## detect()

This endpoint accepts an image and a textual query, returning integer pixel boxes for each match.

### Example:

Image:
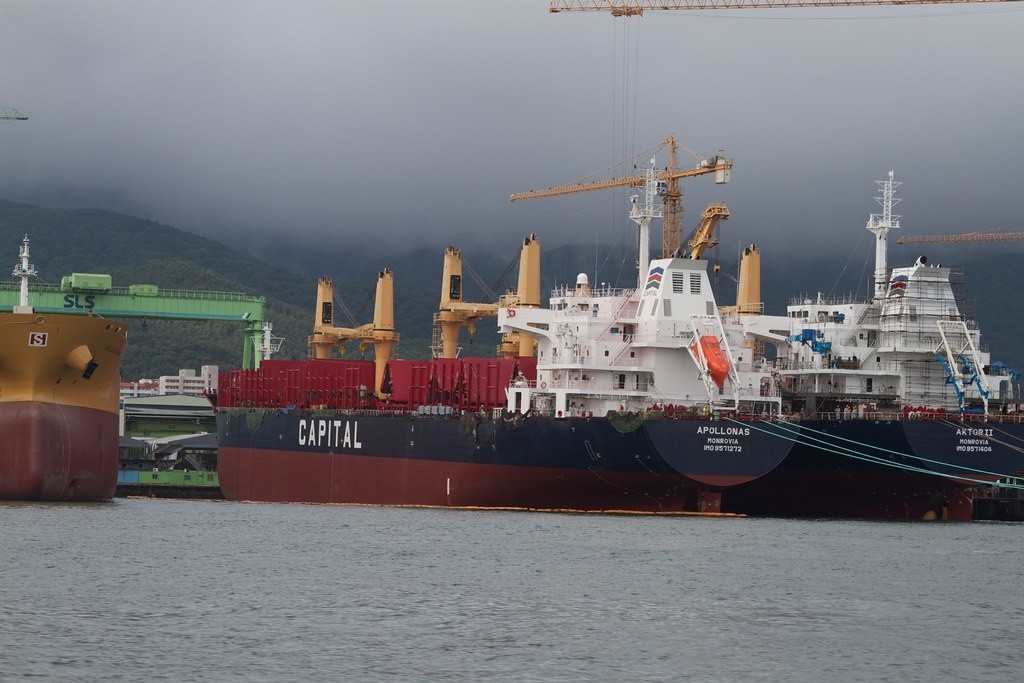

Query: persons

[760,404,858,420]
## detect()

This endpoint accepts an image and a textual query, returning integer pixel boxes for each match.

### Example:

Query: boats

[212,169,1024,530]
[0,234,124,507]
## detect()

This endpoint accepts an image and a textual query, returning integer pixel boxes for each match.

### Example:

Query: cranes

[503,127,767,313]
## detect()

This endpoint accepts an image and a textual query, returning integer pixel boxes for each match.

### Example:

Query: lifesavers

[508,309,516,317]
[540,381,547,389]
